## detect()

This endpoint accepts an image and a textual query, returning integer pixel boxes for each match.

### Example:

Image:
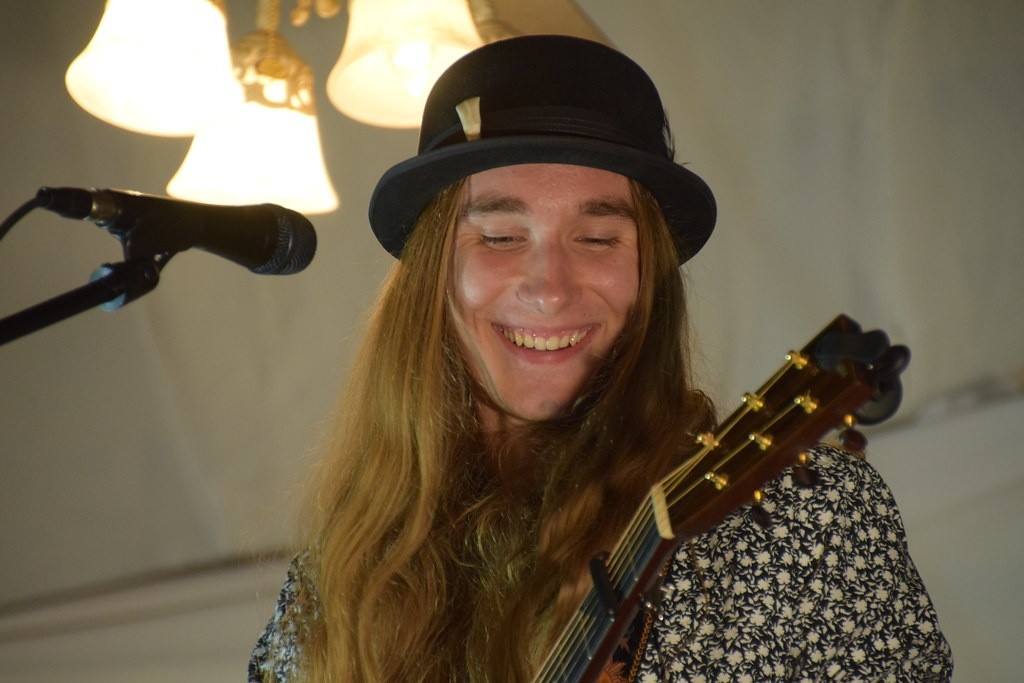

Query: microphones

[36,185,318,275]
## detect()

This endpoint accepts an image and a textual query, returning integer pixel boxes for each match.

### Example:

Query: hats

[366,35,717,266]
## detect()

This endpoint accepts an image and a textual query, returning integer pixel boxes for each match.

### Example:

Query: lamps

[62,0,508,214]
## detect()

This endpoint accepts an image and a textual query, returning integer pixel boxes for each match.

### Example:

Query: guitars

[520,313,909,683]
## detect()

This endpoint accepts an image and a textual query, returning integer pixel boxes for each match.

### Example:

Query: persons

[248,35,955,682]
